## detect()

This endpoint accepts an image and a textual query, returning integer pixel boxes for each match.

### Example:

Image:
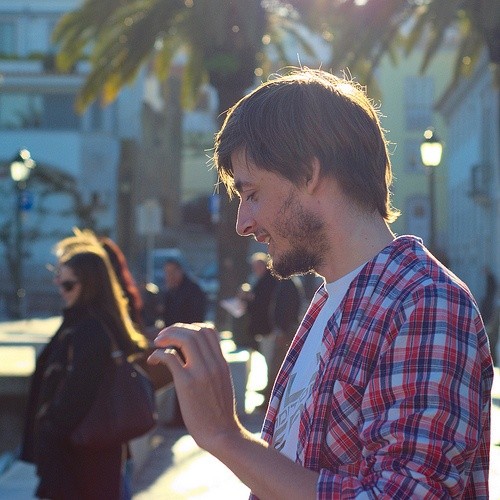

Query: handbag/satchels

[79,351,154,453]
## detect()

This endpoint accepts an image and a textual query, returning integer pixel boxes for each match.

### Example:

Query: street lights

[9,148,36,319]
[420,127,443,261]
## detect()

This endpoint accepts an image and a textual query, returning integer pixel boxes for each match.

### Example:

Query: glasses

[58,280,78,293]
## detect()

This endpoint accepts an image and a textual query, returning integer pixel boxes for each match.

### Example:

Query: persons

[146,72,494,500]
[22,227,310,500]
